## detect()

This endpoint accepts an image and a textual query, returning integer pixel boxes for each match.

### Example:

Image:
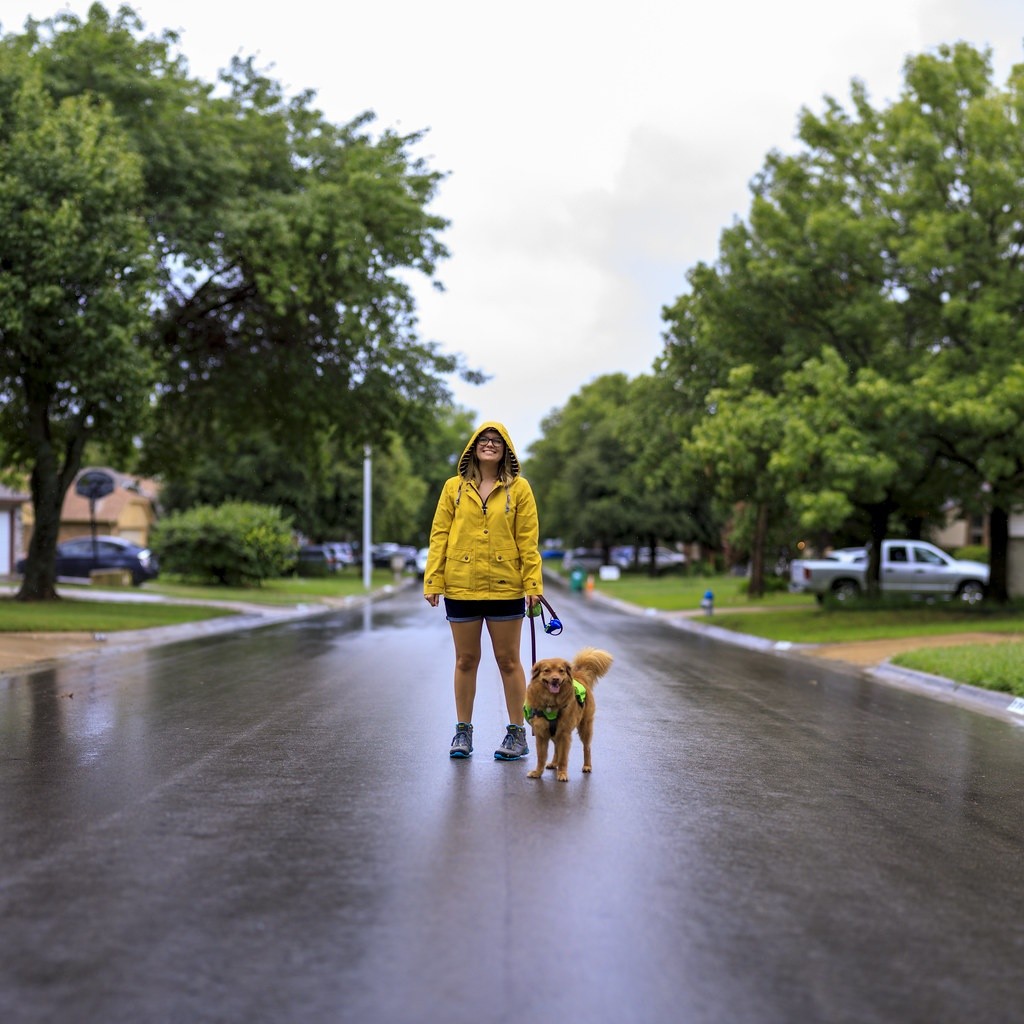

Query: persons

[424,422,542,759]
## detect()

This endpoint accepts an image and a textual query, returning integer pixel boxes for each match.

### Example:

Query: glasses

[475,436,503,447]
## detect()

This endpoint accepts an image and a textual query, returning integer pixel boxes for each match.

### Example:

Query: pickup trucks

[790,537,993,610]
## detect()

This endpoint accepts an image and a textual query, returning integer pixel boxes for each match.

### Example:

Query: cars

[822,547,870,564]
[561,545,686,578]
[297,540,433,582]
[17,534,159,589]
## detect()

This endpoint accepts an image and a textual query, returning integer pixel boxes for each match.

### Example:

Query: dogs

[522,645,614,783]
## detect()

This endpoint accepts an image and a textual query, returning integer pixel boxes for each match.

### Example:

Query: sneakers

[449,722,473,757]
[494,723,529,760]
